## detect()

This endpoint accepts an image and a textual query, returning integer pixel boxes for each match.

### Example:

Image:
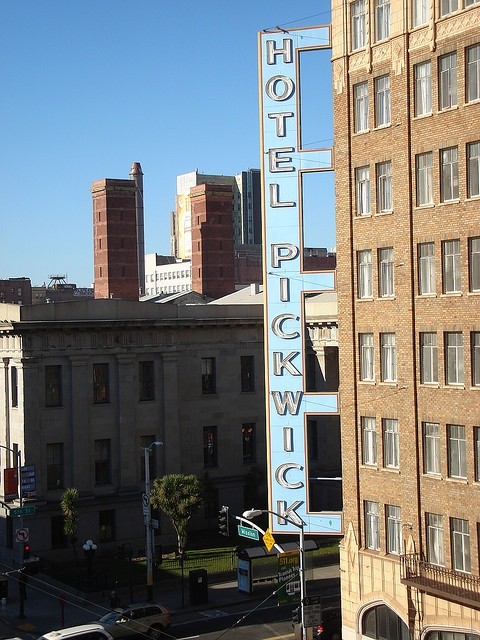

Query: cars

[91,603,171,637]
[36,624,113,640]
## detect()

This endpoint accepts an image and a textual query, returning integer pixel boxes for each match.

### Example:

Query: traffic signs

[302,595,321,626]
[150,519,158,530]
[142,493,150,515]
[15,528,29,542]
[238,525,259,540]
[263,528,276,551]
[10,506,35,517]
[20,465,36,492]
[4,467,18,502]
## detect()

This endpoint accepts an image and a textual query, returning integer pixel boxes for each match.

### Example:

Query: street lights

[144,441,163,602]
[83,540,96,589]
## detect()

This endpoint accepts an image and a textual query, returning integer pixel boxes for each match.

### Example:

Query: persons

[0,591,8,616]
[107,589,121,611]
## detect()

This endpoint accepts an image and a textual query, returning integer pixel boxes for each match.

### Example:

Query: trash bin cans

[189,569,208,606]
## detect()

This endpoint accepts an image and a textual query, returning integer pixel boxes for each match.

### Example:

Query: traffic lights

[24,546,31,559]
[218,506,229,536]
[291,607,301,627]
